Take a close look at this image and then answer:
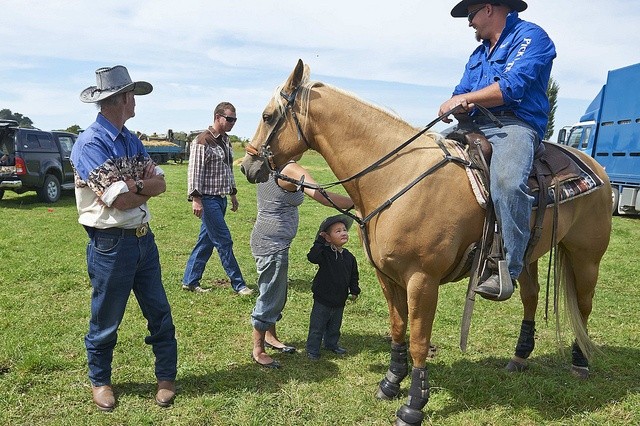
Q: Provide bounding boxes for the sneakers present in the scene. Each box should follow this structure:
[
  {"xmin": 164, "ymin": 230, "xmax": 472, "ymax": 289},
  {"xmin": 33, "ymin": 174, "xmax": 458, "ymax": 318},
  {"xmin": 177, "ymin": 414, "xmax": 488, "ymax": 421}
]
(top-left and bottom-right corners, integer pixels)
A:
[
  {"xmin": 183, "ymin": 285, "xmax": 207, "ymax": 294},
  {"xmin": 237, "ymin": 286, "xmax": 253, "ymax": 295}
]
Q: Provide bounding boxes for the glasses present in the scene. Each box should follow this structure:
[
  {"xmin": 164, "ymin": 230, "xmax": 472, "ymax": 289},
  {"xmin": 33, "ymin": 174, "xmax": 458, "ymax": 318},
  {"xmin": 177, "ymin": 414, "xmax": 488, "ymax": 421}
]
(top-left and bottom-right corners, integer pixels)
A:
[
  {"xmin": 467, "ymin": 4, "xmax": 499, "ymax": 23},
  {"xmin": 218, "ymin": 114, "xmax": 237, "ymax": 122}
]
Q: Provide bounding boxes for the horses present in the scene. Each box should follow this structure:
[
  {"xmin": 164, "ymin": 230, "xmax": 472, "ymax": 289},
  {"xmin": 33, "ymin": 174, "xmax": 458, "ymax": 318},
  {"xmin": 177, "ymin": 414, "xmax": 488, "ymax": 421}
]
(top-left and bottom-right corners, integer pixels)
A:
[{"xmin": 240, "ymin": 58, "xmax": 615, "ymax": 425}]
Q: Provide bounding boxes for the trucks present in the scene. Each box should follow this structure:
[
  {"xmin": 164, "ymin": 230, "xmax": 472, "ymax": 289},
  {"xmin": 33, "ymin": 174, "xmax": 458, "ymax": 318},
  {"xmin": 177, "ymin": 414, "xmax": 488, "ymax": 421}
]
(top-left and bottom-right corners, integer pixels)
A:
[
  {"xmin": 557, "ymin": 63, "xmax": 640, "ymax": 217},
  {"xmin": 144, "ymin": 146, "xmax": 183, "ymax": 165}
]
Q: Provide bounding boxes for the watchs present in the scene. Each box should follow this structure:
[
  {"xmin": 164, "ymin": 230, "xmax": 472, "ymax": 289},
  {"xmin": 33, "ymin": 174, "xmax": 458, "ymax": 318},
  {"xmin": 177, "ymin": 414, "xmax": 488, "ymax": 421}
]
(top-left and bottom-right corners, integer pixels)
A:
[{"xmin": 136, "ymin": 180, "xmax": 144, "ymax": 193}]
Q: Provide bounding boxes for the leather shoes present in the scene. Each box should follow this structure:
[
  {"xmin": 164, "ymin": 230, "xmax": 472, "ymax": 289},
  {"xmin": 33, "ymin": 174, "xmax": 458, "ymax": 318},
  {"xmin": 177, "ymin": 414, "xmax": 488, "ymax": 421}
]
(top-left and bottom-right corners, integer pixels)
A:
[
  {"xmin": 155, "ymin": 379, "xmax": 176, "ymax": 406},
  {"xmin": 474, "ymin": 273, "xmax": 515, "ymax": 297},
  {"xmin": 264, "ymin": 341, "xmax": 295, "ymax": 353},
  {"xmin": 331, "ymin": 346, "xmax": 346, "ymax": 354},
  {"xmin": 91, "ymin": 382, "xmax": 115, "ymax": 411},
  {"xmin": 307, "ymin": 352, "xmax": 321, "ymax": 360},
  {"xmin": 251, "ymin": 352, "xmax": 280, "ymax": 369}
]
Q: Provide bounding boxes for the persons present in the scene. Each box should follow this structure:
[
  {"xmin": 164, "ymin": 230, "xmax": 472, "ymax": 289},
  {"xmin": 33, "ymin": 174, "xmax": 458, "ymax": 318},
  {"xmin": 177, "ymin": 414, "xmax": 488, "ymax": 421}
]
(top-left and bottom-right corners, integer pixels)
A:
[
  {"xmin": 182, "ymin": 102, "xmax": 255, "ymax": 296},
  {"xmin": 250, "ymin": 151, "xmax": 354, "ymax": 368},
  {"xmin": 70, "ymin": 65, "xmax": 178, "ymax": 411},
  {"xmin": 438, "ymin": 0, "xmax": 557, "ymax": 297},
  {"xmin": 306, "ymin": 214, "xmax": 361, "ymax": 359}
]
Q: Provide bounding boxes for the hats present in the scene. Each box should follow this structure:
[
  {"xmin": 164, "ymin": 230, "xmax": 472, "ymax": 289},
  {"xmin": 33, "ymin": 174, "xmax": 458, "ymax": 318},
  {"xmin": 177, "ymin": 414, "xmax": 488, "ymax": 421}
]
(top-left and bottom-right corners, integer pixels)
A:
[
  {"xmin": 79, "ymin": 64, "xmax": 153, "ymax": 103},
  {"xmin": 315, "ymin": 214, "xmax": 353, "ymax": 247},
  {"xmin": 451, "ymin": 0, "xmax": 527, "ymax": 17}
]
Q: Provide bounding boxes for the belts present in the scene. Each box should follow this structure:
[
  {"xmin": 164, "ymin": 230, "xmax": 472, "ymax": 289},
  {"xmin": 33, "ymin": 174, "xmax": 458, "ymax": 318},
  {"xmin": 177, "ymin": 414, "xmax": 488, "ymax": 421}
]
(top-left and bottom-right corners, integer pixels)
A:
[
  {"xmin": 86, "ymin": 222, "xmax": 149, "ymax": 238},
  {"xmin": 202, "ymin": 194, "xmax": 226, "ymax": 198},
  {"xmin": 476, "ymin": 111, "xmax": 516, "ymax": 116}
]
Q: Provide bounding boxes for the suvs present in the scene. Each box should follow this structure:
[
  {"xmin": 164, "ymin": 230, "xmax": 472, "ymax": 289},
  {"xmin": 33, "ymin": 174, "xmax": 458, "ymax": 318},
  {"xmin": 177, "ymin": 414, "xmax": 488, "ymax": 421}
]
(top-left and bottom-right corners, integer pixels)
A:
[{"xmin": 1, "ymin": 119, "xmax": 78, "ymax": 202}]
